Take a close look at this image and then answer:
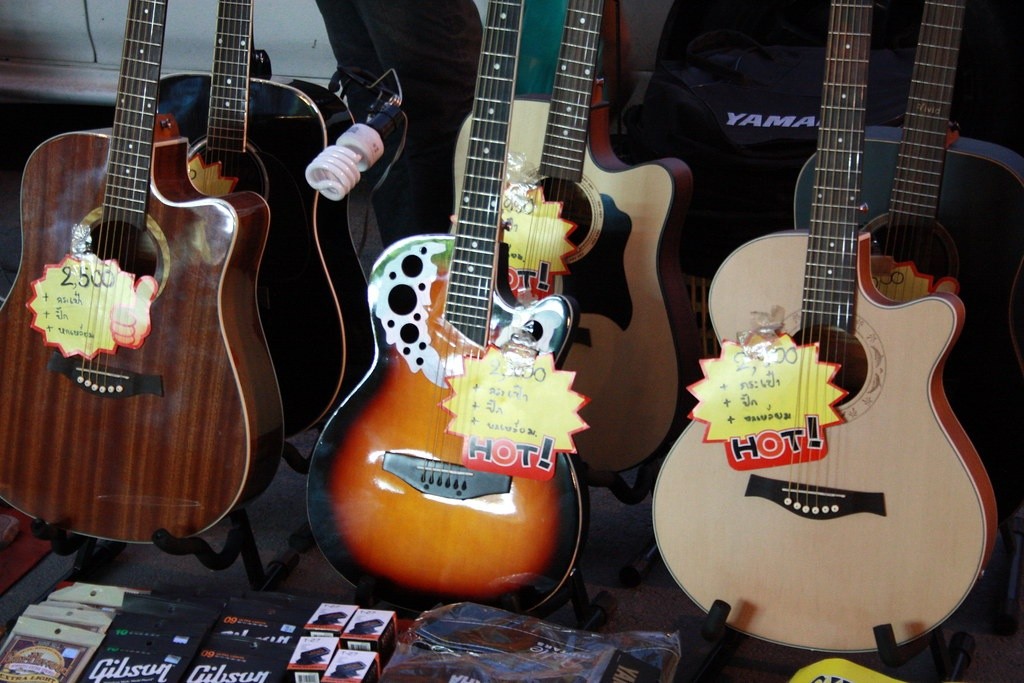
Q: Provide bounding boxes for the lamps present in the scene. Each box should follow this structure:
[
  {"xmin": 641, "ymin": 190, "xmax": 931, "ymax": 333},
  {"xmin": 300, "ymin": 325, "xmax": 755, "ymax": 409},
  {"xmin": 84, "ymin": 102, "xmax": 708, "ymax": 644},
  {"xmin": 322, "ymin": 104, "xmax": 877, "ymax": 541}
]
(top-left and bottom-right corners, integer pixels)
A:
[{"xmin": 305, "ymin": 73, "xmax": 400, "ymax": 201}]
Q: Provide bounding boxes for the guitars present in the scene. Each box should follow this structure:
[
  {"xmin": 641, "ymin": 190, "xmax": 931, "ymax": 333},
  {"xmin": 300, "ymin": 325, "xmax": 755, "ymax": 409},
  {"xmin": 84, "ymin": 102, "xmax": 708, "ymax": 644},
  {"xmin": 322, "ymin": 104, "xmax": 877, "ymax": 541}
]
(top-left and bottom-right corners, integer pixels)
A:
[
  {"xmin": 0, "ymin": 2, "xmax": 287, "ymax": 548},
  {"xmin": 789, "ymin": 1, "xmax": 1023, "ymax": 522},
  {"xmin": 303, "ymin": 0, "xmax": 590, "ymax": 617},
  {"xmin": 449, "ymin": 0, "xmax": 706, "ymax": 473},
  {"xmin": 649, "ymin": 0, "xmax": 1001, "ymax": 655},
  {"xmin": 158, "ymin": 1, "xmax": 376, "ymax": 443}
]
